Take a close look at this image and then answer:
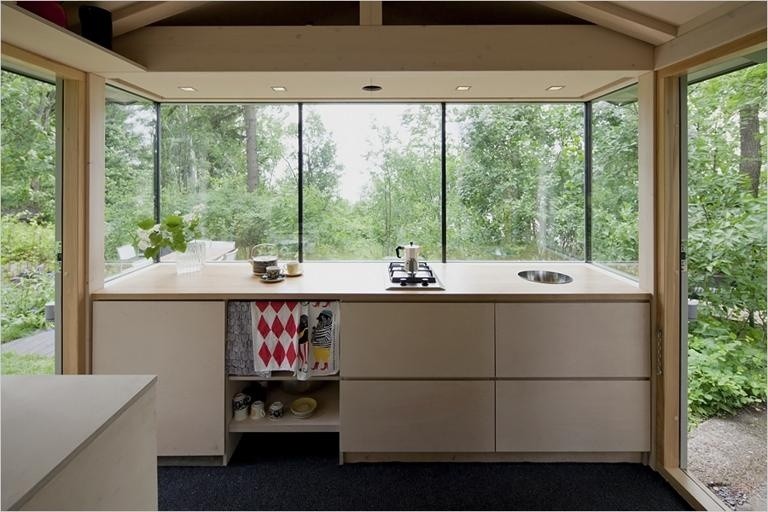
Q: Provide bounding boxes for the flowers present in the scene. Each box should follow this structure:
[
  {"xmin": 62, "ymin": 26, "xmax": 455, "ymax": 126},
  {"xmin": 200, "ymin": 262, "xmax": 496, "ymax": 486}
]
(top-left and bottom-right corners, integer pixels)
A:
[{"xmin": 135, "ymin": 204, "xmax": 209, "ymax": 264}]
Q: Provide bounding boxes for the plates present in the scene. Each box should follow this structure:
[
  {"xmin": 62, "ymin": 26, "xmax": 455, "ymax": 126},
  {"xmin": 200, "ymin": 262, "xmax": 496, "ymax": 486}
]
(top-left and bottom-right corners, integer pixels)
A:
[
  {"xmin": 279, "ymin": 268, "xmax": 304, "ymax": 276},
  {"xmin": 259, "ymin": 277, "xmax": 284, "ymax": 283},
  {"xmin": 288, "ymin": 410, "xmax": 316, "ymax": 419}
]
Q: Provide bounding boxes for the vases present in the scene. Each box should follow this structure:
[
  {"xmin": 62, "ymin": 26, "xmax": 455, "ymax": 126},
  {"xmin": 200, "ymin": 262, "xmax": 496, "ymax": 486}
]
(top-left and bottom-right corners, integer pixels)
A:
[{"xmin": 170, "ymin": 247, "xmax": 203, "ymax": 274}]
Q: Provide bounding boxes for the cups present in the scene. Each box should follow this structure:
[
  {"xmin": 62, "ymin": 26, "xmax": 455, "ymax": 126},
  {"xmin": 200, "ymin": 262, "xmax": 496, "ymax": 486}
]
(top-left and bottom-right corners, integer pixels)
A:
[
  {"xmin": 233, "ymin": 392, "xmax": 251, "ymax": 408},
  {"xmin": 267, "ymin": 402, "xmax": 286, "ymax": 422},
  {"xmin": 266, "ymin": 265, "xmax": 283, "ymax": 279},
  {"xmin": 233, "ymin": 405, "xmax": 249, "ymax": 422},
  {"xmin": 249, "ymin": 402, "xmax": 266, "ymax": 421},
  {"xmin": 283, "ymin": 261, "xmax": 300, "ymax": 274}
]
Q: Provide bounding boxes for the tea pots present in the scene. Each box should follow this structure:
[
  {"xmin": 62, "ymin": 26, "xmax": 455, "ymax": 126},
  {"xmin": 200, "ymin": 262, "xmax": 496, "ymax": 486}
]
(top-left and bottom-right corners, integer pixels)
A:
[{"xmin": 248, "ymin": 243, "xmax": 279, "ymax": 276}]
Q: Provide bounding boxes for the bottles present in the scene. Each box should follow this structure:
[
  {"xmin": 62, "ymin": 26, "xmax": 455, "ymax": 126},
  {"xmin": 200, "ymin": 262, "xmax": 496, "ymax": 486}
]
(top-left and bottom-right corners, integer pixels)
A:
[{"xmin": 395, "ymin": 242, "xmax": 423, "ymax": 274}]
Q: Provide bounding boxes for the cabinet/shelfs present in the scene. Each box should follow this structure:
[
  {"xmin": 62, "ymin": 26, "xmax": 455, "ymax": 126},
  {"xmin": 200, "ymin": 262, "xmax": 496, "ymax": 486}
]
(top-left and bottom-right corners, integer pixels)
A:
[
  {"xmin": 342, "ymin": 295, "xmax": 495, "ymax": 467},
  {"xmin": 225, "ymin": 294, "xmax": 341, "ymax": 434},
  {"xmin": 92, "ymin": 298, "xmax": 225, "ymax": 470},
  {"xmin": 496, "ymin": 297, "xmax": 653, "ymax": 466}
]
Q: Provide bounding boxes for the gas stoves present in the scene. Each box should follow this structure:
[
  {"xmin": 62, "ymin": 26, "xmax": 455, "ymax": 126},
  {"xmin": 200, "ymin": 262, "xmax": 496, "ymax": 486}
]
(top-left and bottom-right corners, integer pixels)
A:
[{"xmin": 384, "ymin": 258, "xmax": 446, "ymax": 292}]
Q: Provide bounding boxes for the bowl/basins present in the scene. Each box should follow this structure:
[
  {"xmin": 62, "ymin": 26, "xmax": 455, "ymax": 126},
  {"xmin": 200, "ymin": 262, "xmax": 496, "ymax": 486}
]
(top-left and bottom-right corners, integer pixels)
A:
[{"xmin": 290, "ymin": 397, "xmax": 318, "ymax": 419}]
